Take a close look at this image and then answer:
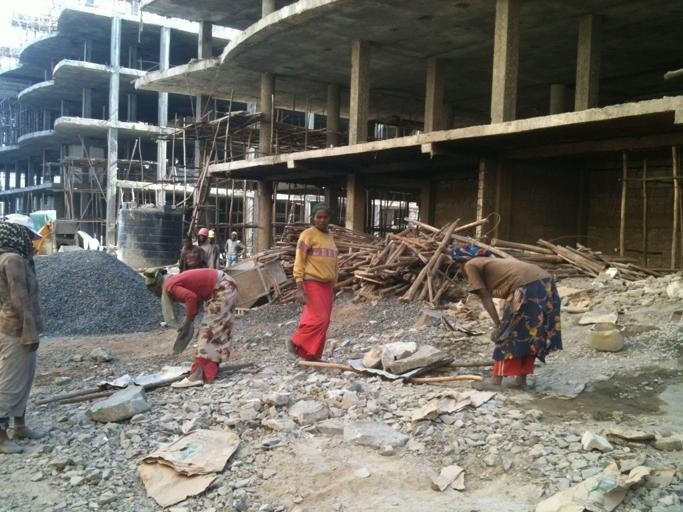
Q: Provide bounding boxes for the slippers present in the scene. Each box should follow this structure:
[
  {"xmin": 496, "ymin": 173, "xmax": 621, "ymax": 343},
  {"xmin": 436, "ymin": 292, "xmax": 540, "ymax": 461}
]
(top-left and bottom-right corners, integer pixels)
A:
[{"xmin": 170, "ymin": 377, "xmax": 203, "ymax": 388}]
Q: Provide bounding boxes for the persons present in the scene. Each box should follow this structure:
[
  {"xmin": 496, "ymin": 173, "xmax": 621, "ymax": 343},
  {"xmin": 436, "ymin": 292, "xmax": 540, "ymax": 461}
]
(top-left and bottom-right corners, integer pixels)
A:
[
  {"xmin": 144, "ymin": 267, "xmax": 238, "ymax": 388},
  {"xmin": 208, "ymin": 229, "xmax": 220, "ymax": 269},
  {"xmin": 284, "ymin": 202, "xmax": 339, "ymax": 363},
  {"xmin": 224, "ymin": 231, "xmax": 245, "ymax": 268},
  {"xmin": 0, "ymin": 213, "xmax": 46, "ymax": 454},
  {"xmin": 450, "ymin": 243, "xmax": 563, "ymax": 393},
  {"xmin": 178, "ymin": 235, "xmax": 208, "ymax": 273},
  {"xmin": 193, "ymin": 228, "xmax": 215, "ymax": 269}
]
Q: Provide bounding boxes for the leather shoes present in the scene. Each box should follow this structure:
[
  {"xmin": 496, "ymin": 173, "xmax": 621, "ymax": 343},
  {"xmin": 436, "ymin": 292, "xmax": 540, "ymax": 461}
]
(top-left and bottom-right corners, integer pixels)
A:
[
  {"xmin": 0, "ymin": 443, "xmax": 23, "ymax": 454},
  {"xmin": 14, "ymin": 429, "xmax": 44, "ymax": 439},
  {"xmin": 285, "ymin": 336, "xmax": 296, "ymax": 360}
]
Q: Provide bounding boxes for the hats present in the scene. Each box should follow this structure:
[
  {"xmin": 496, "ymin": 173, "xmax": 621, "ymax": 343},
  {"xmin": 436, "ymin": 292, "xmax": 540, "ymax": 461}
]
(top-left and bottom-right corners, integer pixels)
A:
[{"xmin": 4, "ymin": 213, "xmax": 42, "ymax": 240}]
